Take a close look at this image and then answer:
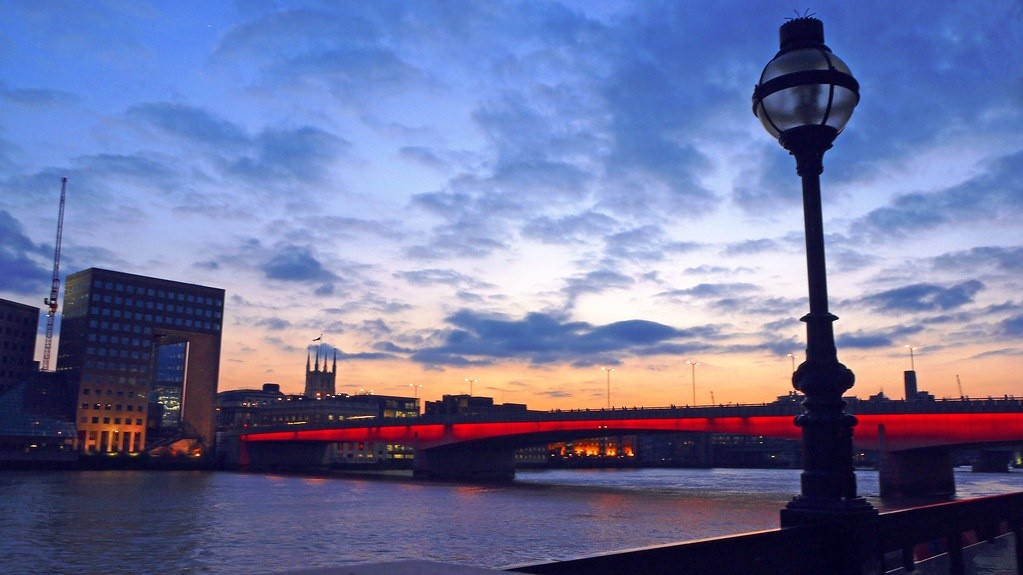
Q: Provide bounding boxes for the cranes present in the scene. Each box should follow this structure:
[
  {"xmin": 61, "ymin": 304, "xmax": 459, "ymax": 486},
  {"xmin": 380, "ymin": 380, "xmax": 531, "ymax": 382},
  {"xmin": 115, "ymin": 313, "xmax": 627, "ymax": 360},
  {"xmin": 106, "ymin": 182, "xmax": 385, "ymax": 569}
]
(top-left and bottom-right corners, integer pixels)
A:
[{"xmin": 41, "ymin": 176, "xmax": 68, "ymax": 370}]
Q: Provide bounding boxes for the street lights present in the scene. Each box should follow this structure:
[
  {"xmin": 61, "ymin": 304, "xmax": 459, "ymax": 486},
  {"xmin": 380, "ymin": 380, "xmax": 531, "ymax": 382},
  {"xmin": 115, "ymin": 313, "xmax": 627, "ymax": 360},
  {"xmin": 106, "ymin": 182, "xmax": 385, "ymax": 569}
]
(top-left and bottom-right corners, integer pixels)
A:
[
  {"xmin": 752, "ymin": 7, "xmax": 881, "ymax": 530},
  {"xmin": 787, "ymin": 353, "xmax": 798, "ymax": 373},
  {"xmin": 464, "ymin": 378, "xmax": 478, "ymax": 396},
  {"xmin": 602, "ymin": 367, "xmax": 615, "ymax": 411},
  {"xmin": 410, "ymin": 384, "xmax": 423, "ymax": 398},
  {"xmin": 686, "ymin": 360, "xmax": 701, "ymax": 408},
  {"xmin": 904, "ymin": 344, "xmax": 918, "ymax": 370}
]
